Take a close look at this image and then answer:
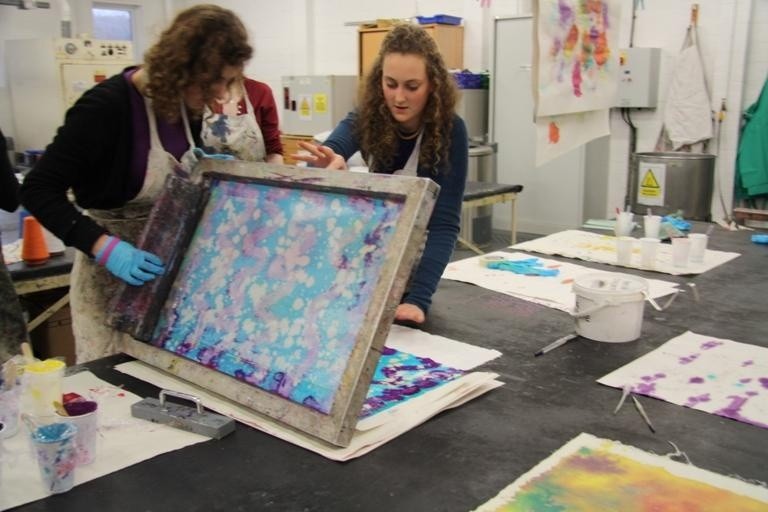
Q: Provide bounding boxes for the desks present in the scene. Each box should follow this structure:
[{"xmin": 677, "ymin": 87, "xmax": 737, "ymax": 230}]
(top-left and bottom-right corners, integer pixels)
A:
[{"xmin": 0, "ymin": 182, "xmax": 768, "ymax": 512}]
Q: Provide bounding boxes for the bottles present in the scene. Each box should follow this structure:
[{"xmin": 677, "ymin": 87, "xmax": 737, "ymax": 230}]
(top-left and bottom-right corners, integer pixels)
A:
[{"xmin": 15, "ymin": 208, "xmax": 65, "ymax": 266}]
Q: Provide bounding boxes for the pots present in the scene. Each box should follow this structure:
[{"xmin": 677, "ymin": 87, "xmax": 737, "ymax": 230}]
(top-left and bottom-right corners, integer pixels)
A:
[{"xmin": 626, "ymin": 150, "xmax": 716, "ymax": 221}]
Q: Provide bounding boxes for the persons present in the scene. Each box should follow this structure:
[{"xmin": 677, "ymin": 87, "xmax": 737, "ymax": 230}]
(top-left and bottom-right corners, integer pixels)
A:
[
  {"xmin": 0, "ymin": 131, "xmax": 22, "ymax": 213},
  {"xmin": 288, "ymin": 23, "xmax": 469, "ymax": 325},
  {"xmin": 198, "ymin": 74, "xmax": 284, "ymax": 166},
  {"xmin": 21, "ymin": 3, "xmax": 255, "ymax": 367}
]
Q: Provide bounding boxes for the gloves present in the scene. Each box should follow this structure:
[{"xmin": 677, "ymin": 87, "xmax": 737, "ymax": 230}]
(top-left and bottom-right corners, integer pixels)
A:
[
  {"xmin": 489, "ymin": 257, "xmax": 559, "ymax": 277},
  {"xmin": 95, "ymin": 238, "xmax": 165, "ymax": 286}
]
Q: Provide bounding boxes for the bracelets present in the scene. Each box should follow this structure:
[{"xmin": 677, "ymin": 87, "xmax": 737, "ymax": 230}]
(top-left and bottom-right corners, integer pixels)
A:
[{"xmin": 97, "ymin": 235, "xmax": 121, "ymax": 266}]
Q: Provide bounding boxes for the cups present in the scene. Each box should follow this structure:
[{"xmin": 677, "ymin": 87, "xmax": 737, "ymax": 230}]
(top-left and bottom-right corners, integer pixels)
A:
[
  {"xmin": 56, "ymin": 399, "xmax": 99, "ymax": 465},
  {"xmin": 642, "ymin": 214, "xmax": 661, "ymax": 239},
  {"xmin": 0, "ymin": 373, "xmax": 24, "ymax": 437},
  {"xmin": 31, "ymin": 421, "xmax": 78, "ymax": 496},
  {"xmin": 669, "ymin": 237, "xmax": 693, "ymax": 269},
  {"xmin": 686, "ymin": 233, "xmax": 709, "ymax": 263},
  {"xmin": 614, "ymin": 235, "xmax": 636, "ymax": 265},
  {"xmin": 23, "ymin": 360, "xmax": 69, "ymax": 417},
  {"xmin": 614, "ymin": 212, "xmax": 634, "ymax": 236},
  {"xmin": 637, "ymin": 237, "xmax": 662, "ymax": 269}
]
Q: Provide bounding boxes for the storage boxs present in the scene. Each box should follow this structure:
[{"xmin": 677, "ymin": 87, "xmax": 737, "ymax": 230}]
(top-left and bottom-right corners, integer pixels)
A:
[{"xmin": 356, "ymin": 25, "xmax": 463, "ymax": 79}]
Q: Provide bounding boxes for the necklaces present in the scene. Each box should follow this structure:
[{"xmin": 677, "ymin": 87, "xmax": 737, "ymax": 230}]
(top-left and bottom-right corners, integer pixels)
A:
[{"xmin": 393, "ymin": 124, "xmax": 422, "ymax": 140}]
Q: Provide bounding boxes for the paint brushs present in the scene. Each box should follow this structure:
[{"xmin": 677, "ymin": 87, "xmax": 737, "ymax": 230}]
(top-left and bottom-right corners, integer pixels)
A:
[
  {"xmin": 631, "ymin": 395, "xmax": 656, "ymax": 434},
  {"xmin": 613, "ymin": 384, "xmax": 634, "ymax": 416},
  {"xmin": 534, "ymin": 333, "xmax": 580, "ymax": 357}
]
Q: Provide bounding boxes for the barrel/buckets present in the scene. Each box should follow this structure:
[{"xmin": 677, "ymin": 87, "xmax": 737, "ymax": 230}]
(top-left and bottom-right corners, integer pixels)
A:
[
  {"xmin": 626, "ymin": 150, "xmax": 719, "ymax": 221},
  {"xmin": 566, "ymin": 275, "xmax": 648, "ymax": 343}
]
